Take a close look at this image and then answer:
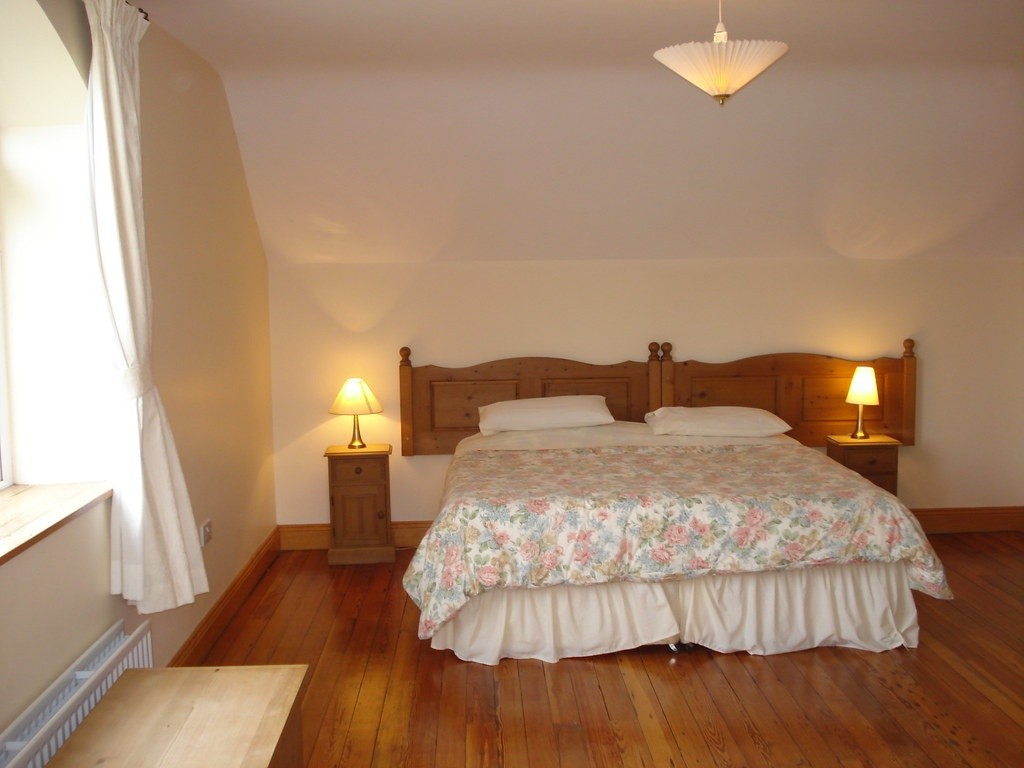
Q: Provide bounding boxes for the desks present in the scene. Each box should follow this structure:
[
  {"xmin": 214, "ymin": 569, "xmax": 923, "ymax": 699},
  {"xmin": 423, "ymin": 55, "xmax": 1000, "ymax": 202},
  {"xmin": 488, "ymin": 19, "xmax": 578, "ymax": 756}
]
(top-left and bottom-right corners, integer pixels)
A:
[{"xmin": 43, "ymin": 662, "xmax": 314, "ymax": 768}]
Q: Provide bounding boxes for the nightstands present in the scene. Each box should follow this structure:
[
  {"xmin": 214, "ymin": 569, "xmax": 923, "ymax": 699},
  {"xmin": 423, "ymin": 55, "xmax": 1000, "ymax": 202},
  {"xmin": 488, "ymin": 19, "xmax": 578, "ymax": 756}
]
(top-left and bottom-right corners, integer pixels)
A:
[
  {"xmin": 824, "ymin": 435, "xmax": 903, "ymax": 496},
  {"xmin": 322, "ymin": 443, "xmax": 395, "ymax": 565}
]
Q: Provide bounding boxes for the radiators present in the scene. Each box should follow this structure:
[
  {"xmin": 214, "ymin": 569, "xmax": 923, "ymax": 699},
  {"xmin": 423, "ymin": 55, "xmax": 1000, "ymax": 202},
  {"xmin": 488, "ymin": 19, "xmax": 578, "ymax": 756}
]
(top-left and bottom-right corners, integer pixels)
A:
[{"xmin": 0, "ymin": 619, "xmax": 154, "ymax": 767}]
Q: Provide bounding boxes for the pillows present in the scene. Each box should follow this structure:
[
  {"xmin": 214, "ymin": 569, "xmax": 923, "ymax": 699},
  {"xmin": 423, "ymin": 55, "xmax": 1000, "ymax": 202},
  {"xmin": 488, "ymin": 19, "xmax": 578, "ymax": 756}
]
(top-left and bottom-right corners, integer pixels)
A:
[
  {"xmin": 644, "ymin": 405, "xmax": 794, "ymax": 436},
  {"xmin": 478, "ymin": 394, "xmax": 615, "ymax": 437}
]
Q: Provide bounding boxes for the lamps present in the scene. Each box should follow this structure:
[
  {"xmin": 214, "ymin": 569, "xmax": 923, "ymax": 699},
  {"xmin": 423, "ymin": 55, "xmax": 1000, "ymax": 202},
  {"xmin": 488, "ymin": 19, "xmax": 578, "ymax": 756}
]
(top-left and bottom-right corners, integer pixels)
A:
[
  {"xmin": 329, "ymin": 377, "xmax": 383, "ymax": 449},
  {"xmin": 843, "ymin": 366, "xmax": 881, "ymax": 439},
  {"xmin": 653, "ymin": 1, "xmax": 791, "ymax": 107}
]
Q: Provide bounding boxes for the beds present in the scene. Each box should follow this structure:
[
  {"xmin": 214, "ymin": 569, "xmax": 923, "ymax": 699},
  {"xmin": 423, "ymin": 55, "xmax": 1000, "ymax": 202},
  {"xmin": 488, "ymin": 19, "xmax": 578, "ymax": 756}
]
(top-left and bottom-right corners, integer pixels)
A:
[{"xmin": 398, "ymin": 338, "xmax": 953, "ymax": 666}]
